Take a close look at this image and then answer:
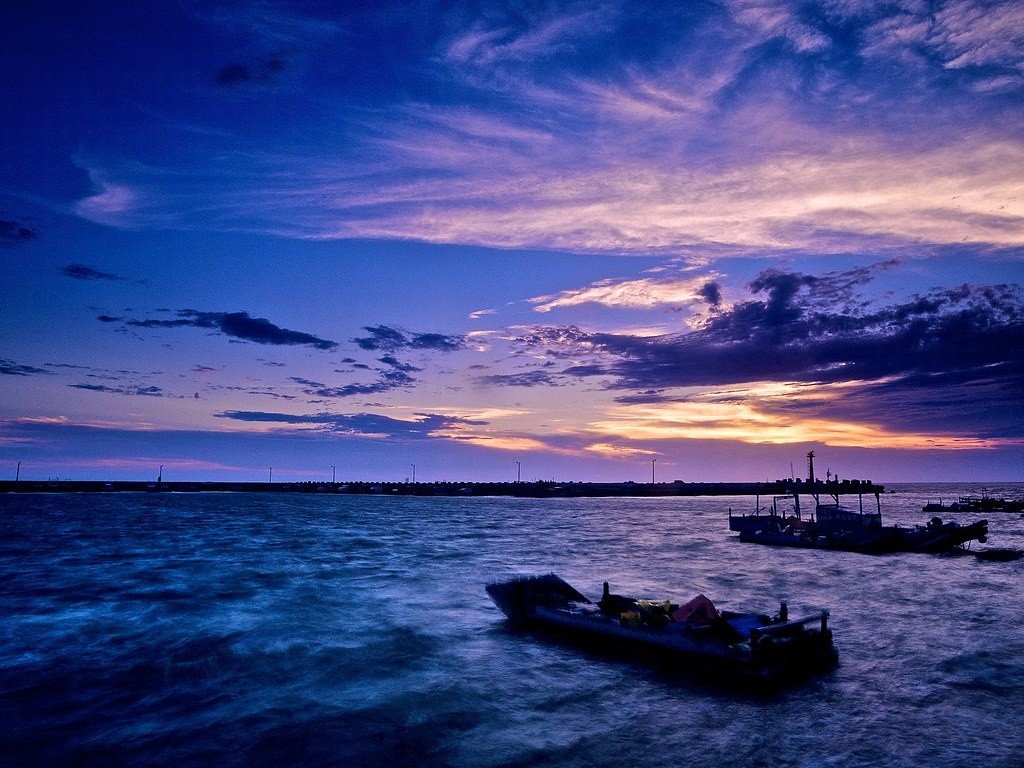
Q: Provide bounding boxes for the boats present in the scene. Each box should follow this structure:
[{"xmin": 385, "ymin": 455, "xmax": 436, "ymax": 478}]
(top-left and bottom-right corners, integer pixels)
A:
[
  {"xmin": 922, "ymin": 492, "xmax": 1024, "ymax": 513},
  {"xmin": 484, "ymin": 571, "xmax": 841, "ymax": 691},
  {"xmin": 728, "ymin": 484, "xmax": 988, "ymax": 556}
]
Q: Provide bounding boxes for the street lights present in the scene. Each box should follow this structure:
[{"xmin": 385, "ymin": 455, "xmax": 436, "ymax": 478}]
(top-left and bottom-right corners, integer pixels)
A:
[
  {"xmin": 159, "ymin": 464, "xmax": 164, "ymax": 482},
  {"xmin": 269, "ymin": 466, "xmax": 272, "ymax": 483},
  {"xmin": 331, "ymin": 465, "xmax": 336, "ymax": 482},
  {"xmin": 652, "ymin": 459, "xmax": 656, "ymax": 483},
  {"xmin": 515, "ymin": 461, "xmax": 521, "ymax": 483},
  {"xmin": 16, "ymin": 462, "xmax": 22, "ymax": 480},
  {"xmin": 411, "ymin": 463, "xmax": 415, "ymax": 483}
]
